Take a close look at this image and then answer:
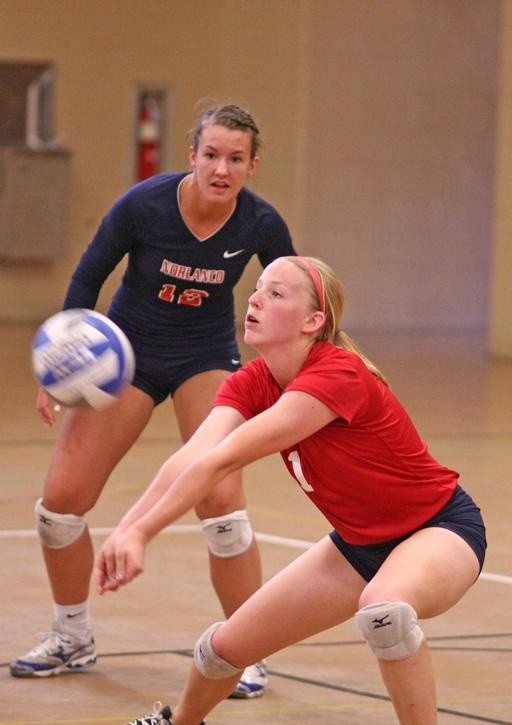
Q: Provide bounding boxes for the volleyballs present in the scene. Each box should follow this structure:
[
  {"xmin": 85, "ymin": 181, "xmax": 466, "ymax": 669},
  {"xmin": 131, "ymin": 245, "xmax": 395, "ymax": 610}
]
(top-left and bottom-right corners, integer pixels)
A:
[{"xmin": 31, "ymin": 308, "xmax": 135, "ymax": 410}]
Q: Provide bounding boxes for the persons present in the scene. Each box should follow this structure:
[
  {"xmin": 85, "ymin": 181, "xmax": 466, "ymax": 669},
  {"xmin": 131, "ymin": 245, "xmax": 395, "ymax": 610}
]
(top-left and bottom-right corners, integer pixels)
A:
[
  {"xmin": 9, "ymin": 105, "xmax": 299, "ymax": 697},
  {"xmin": 94, "ymin": 253, "xmax": 488, "ymax": 725}
]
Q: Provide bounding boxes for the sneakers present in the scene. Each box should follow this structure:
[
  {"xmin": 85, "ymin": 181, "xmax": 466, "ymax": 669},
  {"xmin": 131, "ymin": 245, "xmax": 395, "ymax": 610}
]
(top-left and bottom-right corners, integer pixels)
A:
[
  {"xmin": 9, "ymin": 631, "xmax": 96, "ymax": 678},
  {"xmin": 125, "ymin": 700, "xmax": 206, "ymax": 723},
  {"xmin": 227, "ymin": 658, "xmax": 267, "ymax": 697}
]
